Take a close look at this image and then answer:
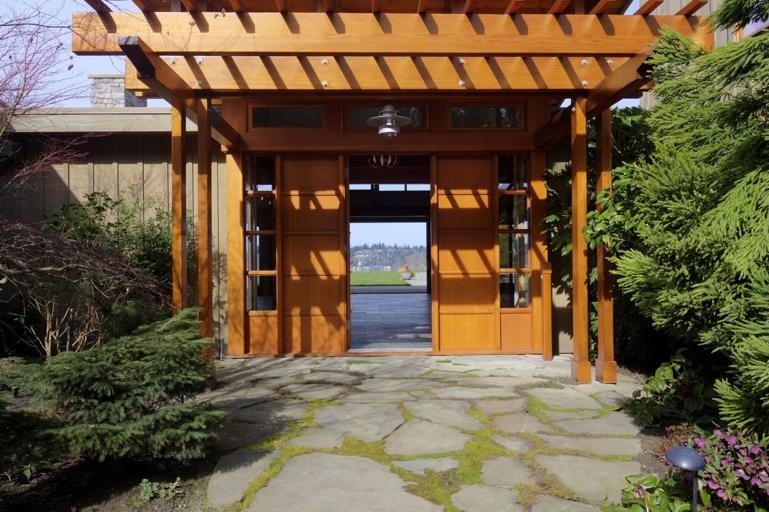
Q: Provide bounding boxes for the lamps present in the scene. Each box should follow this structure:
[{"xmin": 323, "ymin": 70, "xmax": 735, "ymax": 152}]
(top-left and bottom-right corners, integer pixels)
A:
[{"xmin": 365, "ymin": 104, "xmax": 412, "ymax": 138}]
